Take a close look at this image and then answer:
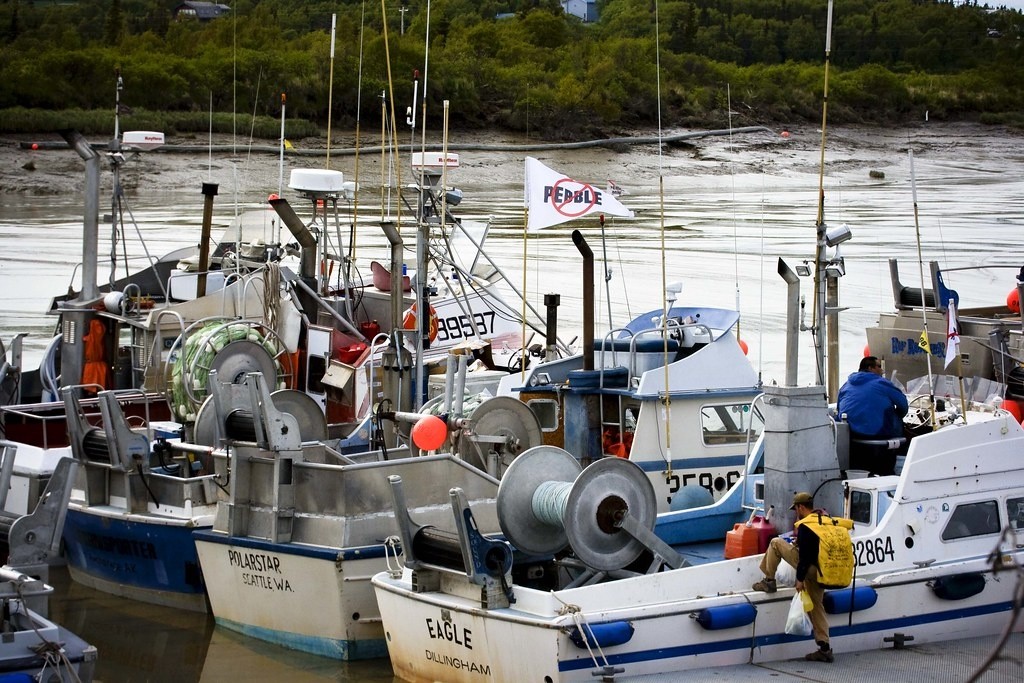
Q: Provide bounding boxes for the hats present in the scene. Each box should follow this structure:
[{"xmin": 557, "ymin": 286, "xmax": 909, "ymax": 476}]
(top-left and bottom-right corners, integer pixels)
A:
[{"xmin": 789, "ymin": 493, "xmax": 813, "ymax": 510}]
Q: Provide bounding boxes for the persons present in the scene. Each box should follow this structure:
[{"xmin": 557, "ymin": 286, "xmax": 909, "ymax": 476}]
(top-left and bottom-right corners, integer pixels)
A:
[
  {"xmin": 838, "ymin": 355, "xmax": 909, "ymax": 477},
  {"xmin": 753, "ymin": 492, "xmax": 835, "ymax": 663}
]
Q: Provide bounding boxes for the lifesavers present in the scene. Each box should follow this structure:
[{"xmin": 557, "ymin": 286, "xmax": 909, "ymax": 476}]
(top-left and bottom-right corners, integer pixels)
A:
[{"xmin": 402, "ymin": 297, "xmax": 440, "ymax": 348}]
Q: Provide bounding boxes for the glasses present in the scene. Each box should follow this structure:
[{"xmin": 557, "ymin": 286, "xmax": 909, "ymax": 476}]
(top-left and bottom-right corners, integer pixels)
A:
[{"xmin": 876, "ymin": 364, "xmax": 881, "ymax": 368}]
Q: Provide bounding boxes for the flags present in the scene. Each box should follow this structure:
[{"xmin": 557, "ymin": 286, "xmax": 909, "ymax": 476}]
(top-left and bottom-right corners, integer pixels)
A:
[
  {"xmin": 606, "ymin": 178, "xmax": 629, "ymax": 197},
  {"xmin": 945, "ymin": 298, "xmax": 960, "ymax": 369},
  {"xmin": 524, "ymin": 159, "xmax": 631, "ymax": 231}
]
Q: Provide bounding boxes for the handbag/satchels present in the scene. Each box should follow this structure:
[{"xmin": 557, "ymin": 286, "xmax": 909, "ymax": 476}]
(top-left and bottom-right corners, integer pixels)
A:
[{"xmin": 784, "ymin": 589, "xmax": 814, "ymax": 636}]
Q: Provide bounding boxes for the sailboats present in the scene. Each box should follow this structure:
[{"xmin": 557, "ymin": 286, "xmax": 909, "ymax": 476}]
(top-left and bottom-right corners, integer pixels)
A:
[{"xmin": 0, "ymin": 1, "xmax": 1024, "ymax": 683}]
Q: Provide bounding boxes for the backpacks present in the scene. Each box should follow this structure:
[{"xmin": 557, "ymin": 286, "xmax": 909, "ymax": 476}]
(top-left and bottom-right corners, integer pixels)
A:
[{"xmin": 794, "ymin": 513, "xmax": 855, "ymax": 589}]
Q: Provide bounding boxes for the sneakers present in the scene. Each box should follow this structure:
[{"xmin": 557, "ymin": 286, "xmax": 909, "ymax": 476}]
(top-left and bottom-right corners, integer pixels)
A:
[
  {"xmin": 752, "ymin": 579, "xmax": 776, "ymax": 593},
  {"xmin": 805, "ymin": 648, "xmax": 834, "ymax": 663}
]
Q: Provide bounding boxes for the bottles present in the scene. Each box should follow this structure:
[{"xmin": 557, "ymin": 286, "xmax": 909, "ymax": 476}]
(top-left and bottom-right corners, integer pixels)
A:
[
  {"xmin": 753, "ymin": 516, "xmax": 777, "ymax": 553},
  {"xmin": 403, "ymin": 264, "xmax": 407, "ymax": 275},
  {"xmin": 725, "ymin": 524, "xmax": 759, "ymax": 559}
]
